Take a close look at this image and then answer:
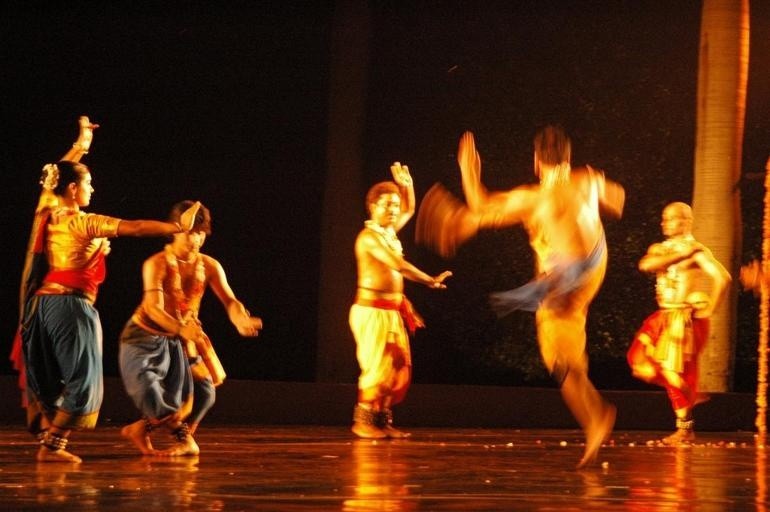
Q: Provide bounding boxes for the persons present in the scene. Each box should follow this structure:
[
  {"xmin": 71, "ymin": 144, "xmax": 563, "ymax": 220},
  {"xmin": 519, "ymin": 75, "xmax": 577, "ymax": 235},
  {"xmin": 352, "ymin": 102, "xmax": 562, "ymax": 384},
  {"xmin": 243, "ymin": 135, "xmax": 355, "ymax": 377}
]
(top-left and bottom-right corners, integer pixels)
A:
[
  {"xmin": 11, "ymin": 116, "xmax": 201, "ymax": 463},
  {"xmin": 403, "ymin": 122, "xmax": 630, "ymax": 471},
  {"xmin": 119, "ymin": 198, "xmax": 264, "ymax": 456},
  {"xmin": 627, "ymin": 200, "xmax": 734, "ymax": 444},
  {"xmin": 348, "ymin": 160, "xmax": 454, "ymax": 440}
]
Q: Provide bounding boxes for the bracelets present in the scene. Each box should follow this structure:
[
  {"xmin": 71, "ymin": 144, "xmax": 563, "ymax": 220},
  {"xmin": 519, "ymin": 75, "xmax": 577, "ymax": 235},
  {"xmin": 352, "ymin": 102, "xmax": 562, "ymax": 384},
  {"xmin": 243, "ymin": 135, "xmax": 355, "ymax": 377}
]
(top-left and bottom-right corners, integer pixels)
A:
[
  {"xmin": 175, "ymin": 221, "xmax": 187, "ymax": 234},
  {"xmin": 72, "ymin": 143, "xmax": 89, "ymax": 155}
]
